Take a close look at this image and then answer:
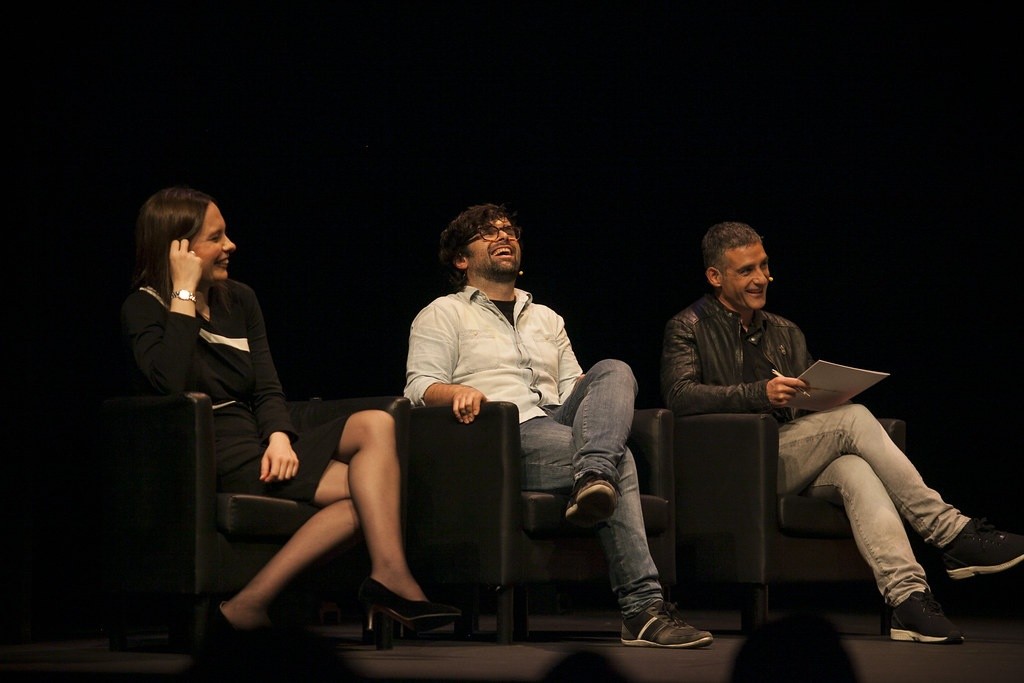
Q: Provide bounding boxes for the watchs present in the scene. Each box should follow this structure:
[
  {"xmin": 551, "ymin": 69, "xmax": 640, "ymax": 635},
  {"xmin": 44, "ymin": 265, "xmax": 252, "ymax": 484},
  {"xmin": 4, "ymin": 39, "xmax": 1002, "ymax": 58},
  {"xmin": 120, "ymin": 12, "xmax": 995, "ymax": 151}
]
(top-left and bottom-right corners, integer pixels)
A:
[{"xmin": 169, "ymin": 288, "xmax": 198, "ymax": 303}]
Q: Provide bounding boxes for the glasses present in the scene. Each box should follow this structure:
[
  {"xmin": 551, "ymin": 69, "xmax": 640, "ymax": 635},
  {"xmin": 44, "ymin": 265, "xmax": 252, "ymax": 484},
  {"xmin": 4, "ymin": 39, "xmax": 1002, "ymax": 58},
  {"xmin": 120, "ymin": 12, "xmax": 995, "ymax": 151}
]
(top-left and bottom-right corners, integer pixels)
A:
[{"xmin": 459, "ymin": 224, "xmax": 522, "ymax": 241}]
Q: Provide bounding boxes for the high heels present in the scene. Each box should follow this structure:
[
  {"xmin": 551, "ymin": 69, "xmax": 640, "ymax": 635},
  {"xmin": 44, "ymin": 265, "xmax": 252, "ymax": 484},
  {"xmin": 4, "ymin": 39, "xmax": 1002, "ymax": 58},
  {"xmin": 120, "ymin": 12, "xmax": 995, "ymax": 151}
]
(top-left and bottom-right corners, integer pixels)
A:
[
  {"xmin": 357, "ymin": 577, "xmax": 464, "ymax": 635},
  {"xmin": 212, "ymin": 602, "xmax": 239, "ymax": 637}
]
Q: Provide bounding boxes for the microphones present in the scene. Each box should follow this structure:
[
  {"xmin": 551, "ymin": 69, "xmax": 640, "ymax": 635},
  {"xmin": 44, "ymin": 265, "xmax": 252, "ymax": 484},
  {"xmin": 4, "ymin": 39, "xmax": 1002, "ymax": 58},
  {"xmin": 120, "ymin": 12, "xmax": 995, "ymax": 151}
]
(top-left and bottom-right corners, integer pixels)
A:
[
  {"xmin": 519, "ymin": 270, "xmax": 523, "ymax": 275},
  {"xmin": 769, "ymin": 277, "xmax": 773, "ymax": 282}
]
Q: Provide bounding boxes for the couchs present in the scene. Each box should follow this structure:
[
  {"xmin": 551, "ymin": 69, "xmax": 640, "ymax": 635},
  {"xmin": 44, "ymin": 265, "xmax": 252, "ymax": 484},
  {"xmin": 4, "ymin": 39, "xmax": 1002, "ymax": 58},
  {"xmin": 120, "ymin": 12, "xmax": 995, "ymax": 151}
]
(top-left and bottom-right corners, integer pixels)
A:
[
  {"xmin": 360, "ymin": 408, "xmax": 680, "ymax": 647},
  {"xmin": 676, "ymin": 413, "xmax": 910, "ymax": 637},
  {"xmin": 106, "ymin": 394, "xmax": 411, "ymax": 652}
]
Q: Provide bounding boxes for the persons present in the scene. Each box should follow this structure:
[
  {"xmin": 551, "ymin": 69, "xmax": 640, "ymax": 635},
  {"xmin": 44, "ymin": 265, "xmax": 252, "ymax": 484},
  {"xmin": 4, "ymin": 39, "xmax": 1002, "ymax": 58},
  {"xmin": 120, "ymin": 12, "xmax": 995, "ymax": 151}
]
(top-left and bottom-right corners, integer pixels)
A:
[
  {"xmin": 526, "ymin": 636, "xmax": 642, "ymax": 683},
  {"xmin": 166, "ymin": 620, "xmax": 395, "ymax": 683},
  {"xmin": 662, "ymin": 221, "xmax": 1024, "ymax": 647},
  {"xmin": 401, "ymin": 201, "xmax": 714, "ymax": 649},
  {"xmin": 108, "ymin": 183, "xmax": 463, "ymax": 662},
  {"xmin": 721, "ymin": 605, "xmax": 868, "ymax": 683}
]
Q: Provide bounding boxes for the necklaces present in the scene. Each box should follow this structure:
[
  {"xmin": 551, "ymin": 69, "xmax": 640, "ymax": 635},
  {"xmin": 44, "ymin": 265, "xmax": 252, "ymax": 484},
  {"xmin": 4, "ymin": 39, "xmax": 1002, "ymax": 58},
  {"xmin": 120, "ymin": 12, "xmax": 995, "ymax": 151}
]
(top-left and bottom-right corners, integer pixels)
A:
[{"xmin": 188, "ymin": 296, "xmax": 213, "ymax": 321}]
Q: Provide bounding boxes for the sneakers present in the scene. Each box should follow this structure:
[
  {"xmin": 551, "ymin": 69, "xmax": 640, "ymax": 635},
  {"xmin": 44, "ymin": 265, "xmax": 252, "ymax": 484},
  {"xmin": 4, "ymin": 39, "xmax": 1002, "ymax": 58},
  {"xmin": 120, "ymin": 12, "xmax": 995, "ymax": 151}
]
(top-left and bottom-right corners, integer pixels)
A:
[
  {"xmin": 888, "ymin": 592, "xmax": 966, "ymax": 644},
  {"xmin": 564, "ymin": 473, "xmax": 617, "ymax": 529},
  {"xmin": 945, "ymin": 518, "xmax": 1024, "ymax": 580},
  {"xmin": 622, "ymin": 601, "xmax": 714, "ymax": 650}
]
